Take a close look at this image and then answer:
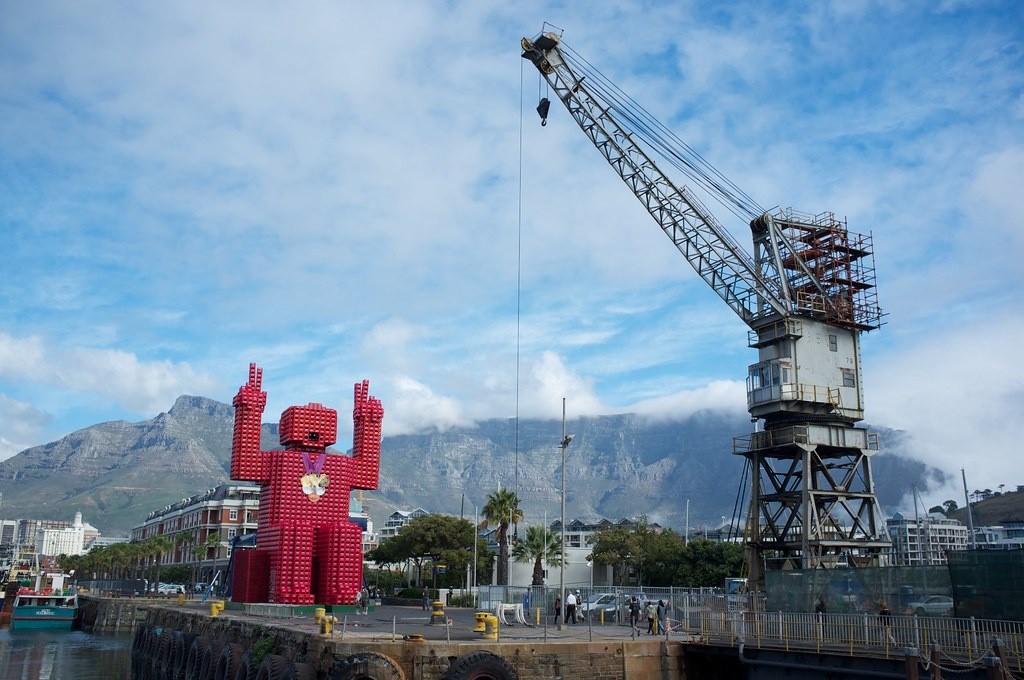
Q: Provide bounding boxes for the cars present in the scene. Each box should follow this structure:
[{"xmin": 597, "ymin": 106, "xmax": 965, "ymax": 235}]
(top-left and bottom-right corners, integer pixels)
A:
[
  {"xmin": 906, "ymin": 594, "xmax": 954, "ymax": 617},
  {"xmin": 579, "ymin": 592, "xmax": 670, "ymax": 623},
  {"xmin": 134, "ymin": 579, "xmax": 210, "ymax": 595}
]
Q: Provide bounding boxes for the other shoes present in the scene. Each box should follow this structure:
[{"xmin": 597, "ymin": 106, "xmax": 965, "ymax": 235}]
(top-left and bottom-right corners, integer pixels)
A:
[
  {"xmin": 663, "ymin": 630, "xmax": 665, "ymax": 635},
  {"xmin": 637, "ymin": 630, "xmax": 640, "ymax": 636},
  {"xmin": 631, "ymin": 634, "xmax": 633, "ymax": 637}
]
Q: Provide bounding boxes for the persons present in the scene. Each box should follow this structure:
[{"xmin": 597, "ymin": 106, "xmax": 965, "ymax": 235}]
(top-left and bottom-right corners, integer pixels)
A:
[
  {"xmin": 354, "ymin": 589, "xmax": 363, "ymax": 616},
  {"xmin": 627, "ymin": 596, "xmax": 640, "ymax": 638},
  {"xmin": 645, "ymin": 601, "xmax": 655, "ymax": 635},
  {"xmin": 657, "ymin": 600, "xmax": 666, "ymax": 635},
  {"xmin": 814, "ymin": 596, "xmax": 826, "ymax": 643},
  {"xmin": 360, "ymin": 587, "xmax": 370, "ymax": 615},
  {"xmin": 877, "ymin": 602, "xmax": 897, "ymax": 646},
  {"xmin": 575, "ymin": 589, "xmax": 584, "ymax": 624},
  {"xmin": 422, "ymin": 586, "xmax": 430, "ymax": 611},
  {"xmin": 184, "ymin": 581, "xmax": 227, "ymax": 600},
  {"xmin": 554, "ymin": 594, "xmax": 560, "ymax": 624},
  {"xmin": 564, "ymin": 590, "xmax": 576, "ymax": 625}
]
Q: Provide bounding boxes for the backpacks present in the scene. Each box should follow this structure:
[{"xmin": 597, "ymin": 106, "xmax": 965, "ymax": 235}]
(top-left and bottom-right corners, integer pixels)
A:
[{"xmin": 576, "ymin": 594, "xmax": 581, "ymax": 605}]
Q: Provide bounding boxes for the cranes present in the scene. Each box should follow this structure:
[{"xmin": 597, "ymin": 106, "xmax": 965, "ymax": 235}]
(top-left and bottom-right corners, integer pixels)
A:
[{"xmin": 517, "ymin": 19, "xmax": 899, "ymax": 594}]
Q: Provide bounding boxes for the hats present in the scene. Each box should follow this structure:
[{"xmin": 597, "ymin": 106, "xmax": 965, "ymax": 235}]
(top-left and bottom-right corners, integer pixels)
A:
[{"xmin": 576, "ymin": 590, "xmax": 580, "ymax": 594}]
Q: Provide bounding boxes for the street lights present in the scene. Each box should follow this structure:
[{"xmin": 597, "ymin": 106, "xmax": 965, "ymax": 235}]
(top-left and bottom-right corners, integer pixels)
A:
[{"xmin": 558, "ymin": 397, "xmax": 578, "ymax": 633}]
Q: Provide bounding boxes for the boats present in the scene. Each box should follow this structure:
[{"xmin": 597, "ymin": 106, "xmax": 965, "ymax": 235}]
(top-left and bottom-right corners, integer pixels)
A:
[{"xmin": 10, "ymin": 591, "xmax": 80, "ymax": 630}]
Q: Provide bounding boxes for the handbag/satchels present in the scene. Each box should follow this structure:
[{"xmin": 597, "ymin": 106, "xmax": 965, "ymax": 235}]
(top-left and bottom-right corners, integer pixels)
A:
[{"xmin": 555, "ymin": 600, "xmax": 560, "ymax": 609}]
